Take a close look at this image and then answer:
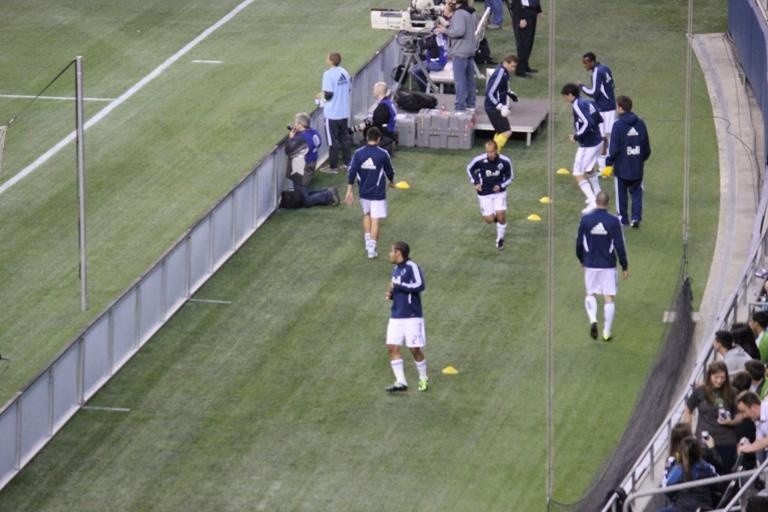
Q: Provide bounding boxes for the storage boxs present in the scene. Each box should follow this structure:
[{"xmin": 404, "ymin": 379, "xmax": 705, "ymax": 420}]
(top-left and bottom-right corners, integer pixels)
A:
[
  {"xmin": 416, "ymin": 108, "xmax": 475, "ymax": 150},
  {"xmin": 367, "ymin": 113, "xmax": 415, "ymax": 147}
]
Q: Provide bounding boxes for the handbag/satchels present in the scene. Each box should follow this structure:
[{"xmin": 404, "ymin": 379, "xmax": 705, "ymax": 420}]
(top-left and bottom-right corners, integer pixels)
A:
[{"xmin": 280, "ymin": 190, "xmax": 303, "ymax": 208}]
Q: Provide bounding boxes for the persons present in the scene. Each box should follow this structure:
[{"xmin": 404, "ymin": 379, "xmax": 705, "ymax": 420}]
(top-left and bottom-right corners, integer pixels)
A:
[
  {"xmin": 561, "ymin": 51, "xmax": 650, "ymax": 229},
  {"xmin": 349, "ymin": 81, "xmax": 397, "ymax": 151},
  {"xmin": 315, "ymin": 53, "xmax": 352, "ymax": 174},
  {"xmin": 410, "ymin": 1, "xmax": 543, "ymax": 113},
  {"xmin": 344, "ymin": 126, "xmax": 395, "ymax": 258},
  {"xmin": 484, "ymin": 55, "xmax": 520, "ymax": 153},
  {"xmin": 385, "ymin": 242, "xmax": 429, "ymax": 391},
  {"xmin": 285, "ymin": 112, "xmax": 340, "ymax": 207},
  {"xmin": 576, "ymin": 191, "xmax": 629, "ymax": 342},
  {"xmin": 466, "ymin": 140, "xmax": 514, "ymax": 250},
  {"xmin": 662, "ymin": 279, "xmax": 768, "ymax": 512}
]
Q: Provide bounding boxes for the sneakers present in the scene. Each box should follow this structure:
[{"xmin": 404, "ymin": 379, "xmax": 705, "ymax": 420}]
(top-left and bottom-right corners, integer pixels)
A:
[
  {"xmin": 590, "ymin": 321, "xmax": 598, "ymax": 340},
  {"xmin": 630, "ymin": 220, "xmax": 639, "ymax": 227},
  {"xmin": 324, "ymin": 186, "xmax": 340, "ymax": 207},
  {"xmin": 496, "ymin": 238, "xmax": 505, "ymax": 250},
  {"xmin": 319, "ymin": 165, "xmax": 349, "ymax": 174},
  {"xmin": 417, "ymin": 377, "xmax": 429, "ymax": 391},
  {"xmin": 385, "ymin": 381, "xmax": 408, "ymax": 392}
]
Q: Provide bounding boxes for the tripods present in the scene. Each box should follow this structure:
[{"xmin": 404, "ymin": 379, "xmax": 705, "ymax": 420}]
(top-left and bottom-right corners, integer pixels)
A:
[{"xmin": 386, "ymin": 51, "xmax": 440, "ymax": 94}]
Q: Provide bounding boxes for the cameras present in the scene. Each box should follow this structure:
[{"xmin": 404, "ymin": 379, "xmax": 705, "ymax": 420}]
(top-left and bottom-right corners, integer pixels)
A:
[{"xmin": 286, "ymin": 123, "xmax": 297, "ymax": 133}]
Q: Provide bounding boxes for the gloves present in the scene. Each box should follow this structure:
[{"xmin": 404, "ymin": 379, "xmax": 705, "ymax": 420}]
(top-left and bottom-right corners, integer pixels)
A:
[
  {"xmin": 509, "ymin": 90, "xmax": 519, "ymax": 102},
  {"xmin": 500, "ymin": 105, "xmax": 511, "ymax": 117}
]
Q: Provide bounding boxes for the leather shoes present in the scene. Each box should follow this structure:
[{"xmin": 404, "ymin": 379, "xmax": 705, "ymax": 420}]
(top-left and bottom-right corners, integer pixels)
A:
[{"xmin": 516, "ymin": 69, "xmax": 538, "ymax": 78}]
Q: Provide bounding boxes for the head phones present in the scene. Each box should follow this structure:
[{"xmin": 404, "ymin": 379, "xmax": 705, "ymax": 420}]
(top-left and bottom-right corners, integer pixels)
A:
[{"xmin": 448, "ymin": 0, "xmax": 461, "ymax": 9}]
